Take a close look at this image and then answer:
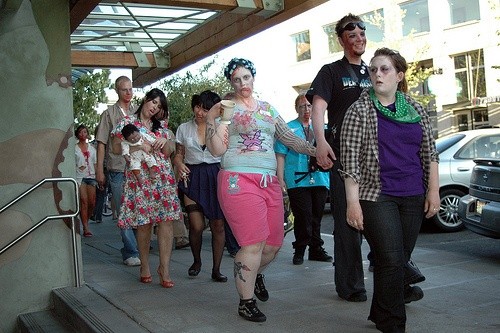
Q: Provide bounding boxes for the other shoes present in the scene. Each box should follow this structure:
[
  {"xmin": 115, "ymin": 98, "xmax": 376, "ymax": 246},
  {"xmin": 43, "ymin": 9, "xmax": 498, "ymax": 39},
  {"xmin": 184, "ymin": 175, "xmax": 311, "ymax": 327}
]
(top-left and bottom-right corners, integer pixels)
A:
[
  {"xmin": 407, "ymin": 260, "xmax": 425, "ymax": 284},
  {"xmin": 123, "ymin": 256, "xmax": 141, "ymax": 266},
  {"xmin": 188, "ymin": 263, "xmax": 201, "ymax": 276},
  {"xmin": 404, "ymin": 281, "xmax": 423, "ymax": 303},
  {"xmin": 83, "ymin": 232, "xmax": 92, "ymax": 237},
  {"xmin": 212, "ymin": 273, "xmax": 227, "ymax": 282},
  {"xmin": 338, "ymin": 290, "xmax": 366, "ymax": 302},
  {"xmin": 308, "ymin": 248, "xmax": 332, "ymax": 262},
  {"xmin": 293, "ymin": 249, "xmax": 304, "ymax": 264}
]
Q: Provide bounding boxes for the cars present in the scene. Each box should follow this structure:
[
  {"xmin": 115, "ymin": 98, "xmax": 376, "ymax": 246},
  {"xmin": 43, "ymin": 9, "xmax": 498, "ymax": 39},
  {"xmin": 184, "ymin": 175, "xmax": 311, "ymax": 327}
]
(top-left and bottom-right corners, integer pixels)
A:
[
  {"xmin": 435, "ymin": 128, "xmax": 498, "ymax": 233},
  {"xmin": 457, "ymin": 156, "xmax": 498, "ymax": 240}
]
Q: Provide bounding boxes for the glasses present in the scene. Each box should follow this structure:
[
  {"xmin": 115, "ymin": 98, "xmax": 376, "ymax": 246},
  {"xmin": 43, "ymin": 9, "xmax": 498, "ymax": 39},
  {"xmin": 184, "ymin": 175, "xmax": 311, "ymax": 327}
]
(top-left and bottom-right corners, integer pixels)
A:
[{"xmin": 341, "ymin": 22, "xmax": 365, "ymax": 36}]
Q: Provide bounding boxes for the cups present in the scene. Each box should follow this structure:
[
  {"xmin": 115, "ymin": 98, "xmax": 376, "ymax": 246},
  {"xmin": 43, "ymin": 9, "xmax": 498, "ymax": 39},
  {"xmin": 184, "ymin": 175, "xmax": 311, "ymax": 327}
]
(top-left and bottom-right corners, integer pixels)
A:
[{"xmin": 218, "ymin": 99, "xmax": 236, "ymax": 125}]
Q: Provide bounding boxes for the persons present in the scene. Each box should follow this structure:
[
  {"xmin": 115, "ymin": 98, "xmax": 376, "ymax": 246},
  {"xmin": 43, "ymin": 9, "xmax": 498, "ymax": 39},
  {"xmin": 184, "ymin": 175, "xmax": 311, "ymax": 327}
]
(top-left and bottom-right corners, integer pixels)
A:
[
  {"xmin": 337, "ymin": 49, "xmax": 441, "ymax": 333},
  {"xmin": 73, "ymin": 125, "xmax": 98, "ymax": 238},
  {"xmin": 120, "ymin": 123, "xmax": 157, "ymax": 180},
  {"xmin": 306, "ymin": 14, "xmax": 424, "ymax": 305},
  {"xmin": 91, "ymin": 76, "xmax": 240, "ymax": 287},
  {"xmin": 205, "ymin": 57, "xmax": 333, "ymax": 323},
  {"xmin": 274, "ymin": 93, "xmax": 333, "ymax": 264}
]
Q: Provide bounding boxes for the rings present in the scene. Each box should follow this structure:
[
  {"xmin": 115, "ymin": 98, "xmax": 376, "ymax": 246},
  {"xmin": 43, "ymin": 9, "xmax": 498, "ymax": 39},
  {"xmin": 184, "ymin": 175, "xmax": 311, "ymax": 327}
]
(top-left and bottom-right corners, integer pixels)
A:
[{"xmin": 433, "ymin": 208, "xmax": 435, "ymax": 210}]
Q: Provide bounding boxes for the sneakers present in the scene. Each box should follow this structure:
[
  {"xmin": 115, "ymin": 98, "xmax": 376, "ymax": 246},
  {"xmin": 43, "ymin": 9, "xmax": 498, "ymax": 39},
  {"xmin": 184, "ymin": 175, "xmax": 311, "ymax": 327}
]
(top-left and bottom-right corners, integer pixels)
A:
[
  {"xmin": 254, "ymin": 274, "xmax": 269, "ymax": 302},
  {"xmin": 238, "ymin": 299, "xmax": 266, "ymax": 322}
]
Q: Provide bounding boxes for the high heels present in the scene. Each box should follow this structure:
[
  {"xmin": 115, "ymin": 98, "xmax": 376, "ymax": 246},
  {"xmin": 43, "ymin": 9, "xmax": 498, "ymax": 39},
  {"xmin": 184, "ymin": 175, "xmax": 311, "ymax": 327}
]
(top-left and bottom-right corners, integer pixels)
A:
[
  {"xmin": 140, "ymin": 267, "xmax": 152, "ymax": 283},
  {"xmin": 157, "ymin": 266, "xmax": 175, "ymax": 287}
]
